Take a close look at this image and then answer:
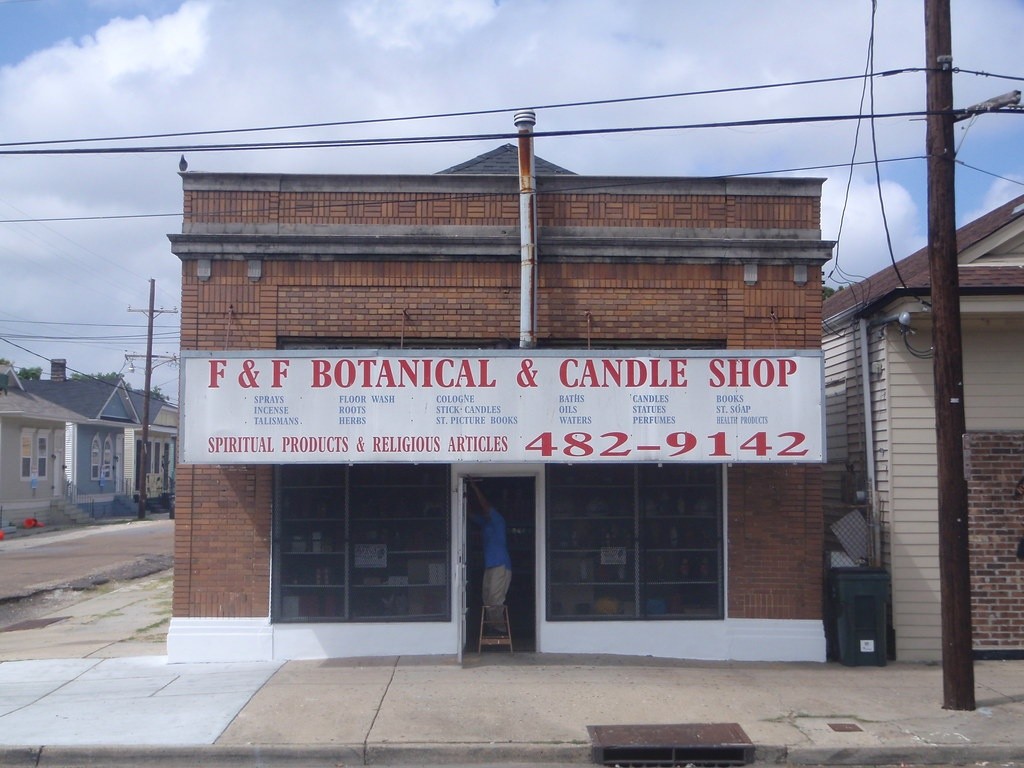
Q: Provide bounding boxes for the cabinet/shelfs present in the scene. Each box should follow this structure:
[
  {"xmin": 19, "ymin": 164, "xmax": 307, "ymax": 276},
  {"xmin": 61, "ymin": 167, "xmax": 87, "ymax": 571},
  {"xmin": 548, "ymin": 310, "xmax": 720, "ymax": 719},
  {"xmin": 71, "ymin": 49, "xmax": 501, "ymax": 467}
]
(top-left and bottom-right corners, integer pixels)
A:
[
  {"xmin": 279, "ymin": 464, "xmax": 451, "ymax": 621},
  {"xmin": 545, "ymin": 463, "xmax": 724, "ymax": 620}
]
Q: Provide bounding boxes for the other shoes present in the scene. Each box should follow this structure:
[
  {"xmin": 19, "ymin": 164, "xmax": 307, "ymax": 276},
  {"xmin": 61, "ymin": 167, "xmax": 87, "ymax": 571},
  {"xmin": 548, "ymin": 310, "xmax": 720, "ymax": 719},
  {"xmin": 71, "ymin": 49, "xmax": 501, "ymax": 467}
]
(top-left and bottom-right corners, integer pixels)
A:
[{"xmin": 483, "ymin": 629, "xmax": 507, "ymax": 637}]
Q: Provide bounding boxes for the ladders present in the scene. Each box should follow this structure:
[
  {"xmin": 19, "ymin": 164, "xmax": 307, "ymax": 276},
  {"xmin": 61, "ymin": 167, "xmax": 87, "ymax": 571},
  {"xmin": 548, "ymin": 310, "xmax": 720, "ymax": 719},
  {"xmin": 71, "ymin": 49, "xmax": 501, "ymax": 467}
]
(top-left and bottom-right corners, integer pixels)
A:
[{"xmin": 475, "ymin": 603, "xmax": 514, "ymax": 654}]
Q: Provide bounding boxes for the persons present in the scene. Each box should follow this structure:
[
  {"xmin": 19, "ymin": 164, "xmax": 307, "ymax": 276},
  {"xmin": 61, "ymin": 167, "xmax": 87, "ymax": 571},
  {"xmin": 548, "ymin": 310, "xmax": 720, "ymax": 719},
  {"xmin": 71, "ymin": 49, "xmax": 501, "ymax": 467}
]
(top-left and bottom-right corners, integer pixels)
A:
[{"xmin": 461, "ymin": 474, "xmax": 512, "ymax": 632}]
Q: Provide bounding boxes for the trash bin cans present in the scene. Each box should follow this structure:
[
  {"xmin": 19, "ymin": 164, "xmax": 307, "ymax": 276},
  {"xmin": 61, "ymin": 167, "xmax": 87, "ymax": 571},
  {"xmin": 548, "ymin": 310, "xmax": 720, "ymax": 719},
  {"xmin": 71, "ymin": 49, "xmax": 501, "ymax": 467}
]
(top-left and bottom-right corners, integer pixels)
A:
[{"xmin": 828, "ymin": 565, "xmax": 891, "ymax": 667}]
